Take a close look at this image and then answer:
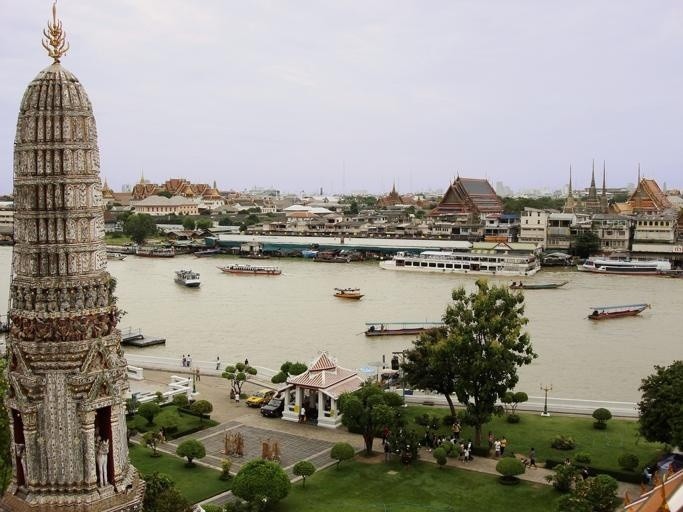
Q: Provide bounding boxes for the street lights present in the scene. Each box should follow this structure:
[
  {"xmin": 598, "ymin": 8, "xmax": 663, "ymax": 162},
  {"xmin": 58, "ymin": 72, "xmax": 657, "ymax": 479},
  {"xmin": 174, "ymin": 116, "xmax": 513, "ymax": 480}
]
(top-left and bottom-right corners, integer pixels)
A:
[
  {"xmin": 537, "ymin": 381, "xmax": 554, "ymax": 418},
  {"xmin": 391, "ymin": 348, "xmax": 414, "ymax": 409}
]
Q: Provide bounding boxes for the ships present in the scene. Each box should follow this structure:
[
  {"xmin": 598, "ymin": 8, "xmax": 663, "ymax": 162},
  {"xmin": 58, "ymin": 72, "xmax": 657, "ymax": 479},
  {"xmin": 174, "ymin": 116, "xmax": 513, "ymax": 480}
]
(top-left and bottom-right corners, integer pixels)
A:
[{"xmin": 377, "ymin": 248, "xmax": 541, "ymax": 278}]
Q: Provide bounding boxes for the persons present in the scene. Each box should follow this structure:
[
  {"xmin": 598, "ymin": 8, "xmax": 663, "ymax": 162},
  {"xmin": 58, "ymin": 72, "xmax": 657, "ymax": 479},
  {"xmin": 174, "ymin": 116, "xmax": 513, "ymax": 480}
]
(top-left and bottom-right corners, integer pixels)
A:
[
  {"xmin": 245, "ymin": 358, "xmax": 249, "ymax": 366},
  {"xmin": 643, "ymin": 466, "xmax": 653, "ymax": 483},
  {"xmin": 289, "ymin": 394, "xmax": 329, "ymax": 424},
  {"xmin": 564, "ymin": 457, "xmax": 570, "ymax": 467},
  {"xmin": 235, "ymin": 392, "xmax": 240, "ymax": 406},
  {"xmin": 216, "ymin": 357, "xmax": 221, "ymax": 370},
  {"xmin": 195, "ymin": 368, "xmax": 201, "ymax": 381},
  {"xmin": 158, "ymin": 426, "xmax": 168, "ymax": 443},
  {"xmin": 582, "ymin": 467, "xmax": 589, "ymax": 479},
  {"xmin": 182, "ymin": 354, "xmax": 187, "ymax": 367},
  {"xmin": 9, "ymin": 284, "xmax": 111, "ymax": 341},
  {"xmin": 382, "ymin": 421, "xmax": 507, "ymax": 463},
  {"xmin": 230, "ymin": 389, "xmax": 235, "ymax": 403},
  {"xmin": 187, "ymin": 354, "xmax": 192, "ymax": 366},
  {"xmin": 527, "ymin": 448, "xmax": 537, "ymax": 469}
]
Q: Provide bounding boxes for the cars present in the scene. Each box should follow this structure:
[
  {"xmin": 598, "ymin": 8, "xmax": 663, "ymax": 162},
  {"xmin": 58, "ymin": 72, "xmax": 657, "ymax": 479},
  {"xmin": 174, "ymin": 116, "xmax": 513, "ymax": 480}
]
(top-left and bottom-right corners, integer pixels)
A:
[
  {"xmin": 244, "ymin": 387, "xmax": 304, "ymax": 419},
  {"xmin": 642, "ymin": 465, "xmax": 656, "ymax": 485}
]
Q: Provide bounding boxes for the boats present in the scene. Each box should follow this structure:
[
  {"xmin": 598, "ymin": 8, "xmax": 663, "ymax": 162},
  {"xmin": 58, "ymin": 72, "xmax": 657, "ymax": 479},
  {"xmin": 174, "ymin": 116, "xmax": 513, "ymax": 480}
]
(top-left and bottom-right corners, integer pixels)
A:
[
  {"xmin": 193, "ymin": 248, "xmax": 222, "ymax": 257},
  {"xmin": 507, "ymin": 279, "xmax": 568, "ymax": 290},
  {"xmin": 0, "ymin": 313, "xmax": 8, "ymax": 334},
  {"xmin": 216, "ymin": 264, "xmax": 282, "ymax": 276},
  {"xmin": 542, "ymin": 252, "xmax": 573, "ymax": 268},
  {"xmin": 173, "ymin": 269, "xmax": 200, "ymax": 289},
  {"xmin": 575, "ymin": 256, "xmax": 672, "ymax": 276},
  {"xmin": 133, "ymin": 245, "xmax": 176, "ymax": 257},
  {"xmin": 586, "ymin": 302, "xmax": 651, "ymax": 320},
  {"xmin": 238, "ymin": 252, "xmax": 269, "ymax": 259},
  {"xmin": 106, "ymin": 251, "xmax": 127, "ymax": 261},
  {"xmin": 312, "ymin": 256, "xmax": 345, "ymax": 263},
  {"xmin": 332, "ymin": 287, "xmax": 363, "ymax": 301},
  {"xmin": 359, "ymin": 319, "xmax": 462, "ymax": 336}
]
